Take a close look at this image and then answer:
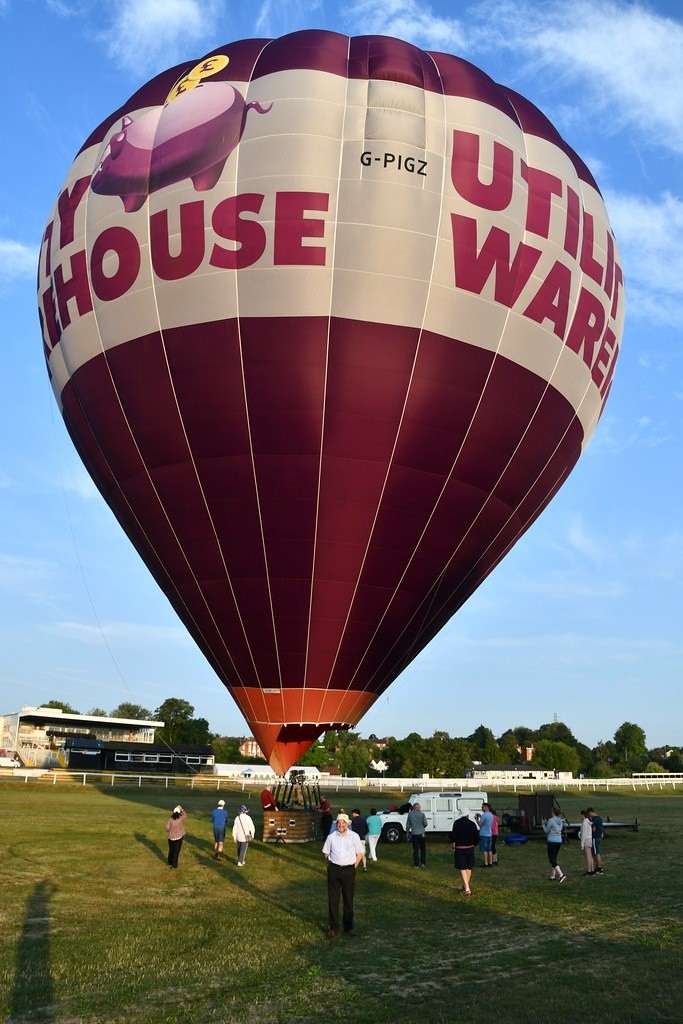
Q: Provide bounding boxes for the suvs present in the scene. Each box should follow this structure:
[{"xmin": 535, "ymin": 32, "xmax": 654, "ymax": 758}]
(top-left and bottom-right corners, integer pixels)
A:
[
  {"xmin": 0, "ymin": 757, "xmax": 21, "ymax": 768},
  {"xmin": 373, "ymin": 791, "xmax": 489, "ymax": 843}
]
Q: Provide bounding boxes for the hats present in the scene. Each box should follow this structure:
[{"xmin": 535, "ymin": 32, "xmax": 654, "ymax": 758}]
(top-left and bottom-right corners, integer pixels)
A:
[
  {"xmin": 332, "ymin": 813, "xmax": 352, "ymax": 826},
  {"xmin": 173, "ymin": 807, "xmax": 180, "ymax": 813},
  {"xmin": 458, "ymin": 806, "xmax": 471, "ymax": 816},
  {"xmin": 237, "ymin": 805, "xmax": 249, "ymax": 814},
  {"xmin": 217, "ymin": 800, "xmax": 225, "ymax": 806}
]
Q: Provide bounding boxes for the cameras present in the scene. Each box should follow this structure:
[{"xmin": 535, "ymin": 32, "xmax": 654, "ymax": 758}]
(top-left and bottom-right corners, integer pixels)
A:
[{"xmin": 475, "ymin": 814, "xmax": 480, "ymax": 819}]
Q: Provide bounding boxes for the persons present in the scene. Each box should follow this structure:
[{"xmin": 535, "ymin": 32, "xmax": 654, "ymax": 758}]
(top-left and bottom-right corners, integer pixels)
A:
[
  {"xmin": 366, "ymin": 808, "xmax": 383, "ymax": 861},
  {"xmin": 350, "ymin": 809, "xmax": 369, "ymax": 871},
  {"xmin": 330, "ymin": 809, "xmax": 351, "ymax": 833},
  {"xmin": 542, "ymin": 808, "xmax": 566, "ymax": 883},
  {"xmin": 282, "ymin": 788, "xmax": 305, "ymax": 809},
  {"xmin": 315, "ymin": 793, "xmax": 333, "ymax": 841},
  {"xmin": 581, "ymin": 810, "xmax": 595, "ymax": 876},
  {"xmin": 165, "ymin": 805, "xmax": 187, "ymax": 870},
  {"xmin": 212, "ymin": 800, "xmax": 228, "ymax": 860},
  {"xmin": 261, "ymin": 785, "xmax": 280, "ymax": 811},
  {"xmin": 588, "ymin": 807, "xmax": 603, "ymax": 874},
  {"xmin": 475, "ymin": 803, "xmax": 498, "ymax": 867},
  {"xmin": 406, "ymin": 803, "xmax": 428, "ymax": 868},
  {"xmin": 322, "ymin": 814, "xmax": 363, "ymax": 937},
  {"xmin": 451, "ymin": 807, "xmax": 480, "ymax": 896},
  {"xmin": 232, "ymin": 805, "xmax": 255, "ymax": 866}
]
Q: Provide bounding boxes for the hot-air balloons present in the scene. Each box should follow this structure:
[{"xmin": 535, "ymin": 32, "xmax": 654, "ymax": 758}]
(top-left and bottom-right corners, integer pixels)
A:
[{"xmin": 37, "ymin": 30, "xmax": 625, "ymax": 843}]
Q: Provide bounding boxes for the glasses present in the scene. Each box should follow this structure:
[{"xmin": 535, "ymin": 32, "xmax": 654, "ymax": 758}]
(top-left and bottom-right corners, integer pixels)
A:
[{"xmin": 482, "ymin": 806, "xmax": 485, "ymax": 808}]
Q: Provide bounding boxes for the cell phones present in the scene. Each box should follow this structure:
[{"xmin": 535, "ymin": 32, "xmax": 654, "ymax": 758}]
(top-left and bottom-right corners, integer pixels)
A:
[{"xmin": 542, "ymin": 816, "xmax": 545, "ymax": 820}]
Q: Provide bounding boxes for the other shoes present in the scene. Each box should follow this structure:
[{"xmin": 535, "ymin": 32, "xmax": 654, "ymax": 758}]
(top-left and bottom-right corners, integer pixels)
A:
[
  {"xmin": 467, "ymin": 892, "xmax": 472, "ymax": 897},
  {"xmin": 351, "ymin": 808, "xmax": 360, "ymax": 814},
  {"xmin": 215, "ymin": 851, "xmax": 223, "ymax": 861},
  {"xmin": 328, "ymin": 929, "xmax": 337, "ymax": 937},
  {"xmin": 593, "ymin": 867, "xmax": 604, "ymax": 874},
  {"xmin": 363, "ymin": 867, "xmax": 367, "ymax": 872},
  {"xmin": 369, "ymin": 857, "xmax": 377, "ymax": 861},
  {"xmin": 558, "ymin": 874, "xmax": 566, "ymax": 883},
  {"xmin": 480, "ymin": 863, "xmax": 488, "ymax": 868},
  {"xmin": 548, "ymin": 876, "xmax": 556, "ymax": 881},
  {"xmin": 344, "ymin": 929, "xmax": 354, "ymax": 936},
  {"xmin": 421, "ymin": 864, "xmax": 426, "ymax": 868},
  {"xmin": 237, "ymin": 861, "xmax": 245, "ymax": 866},
  {"xmin": 413, "ymin": 865, "xmax": 419, "ymax": 869},
  {"xmin": 492, "ymin": 861, "xmax": 498, "ymax": 866},
  {"xmin": 582, "ymin": 870, "xmax": 595, "ymax": 877},
  {"xmin": 488, "ymin": 862, "xmax": 493, "ymax": 868},
  {"xmin": 462, "ymin": 885, "xmax": 465, "ymax": 890}
]
